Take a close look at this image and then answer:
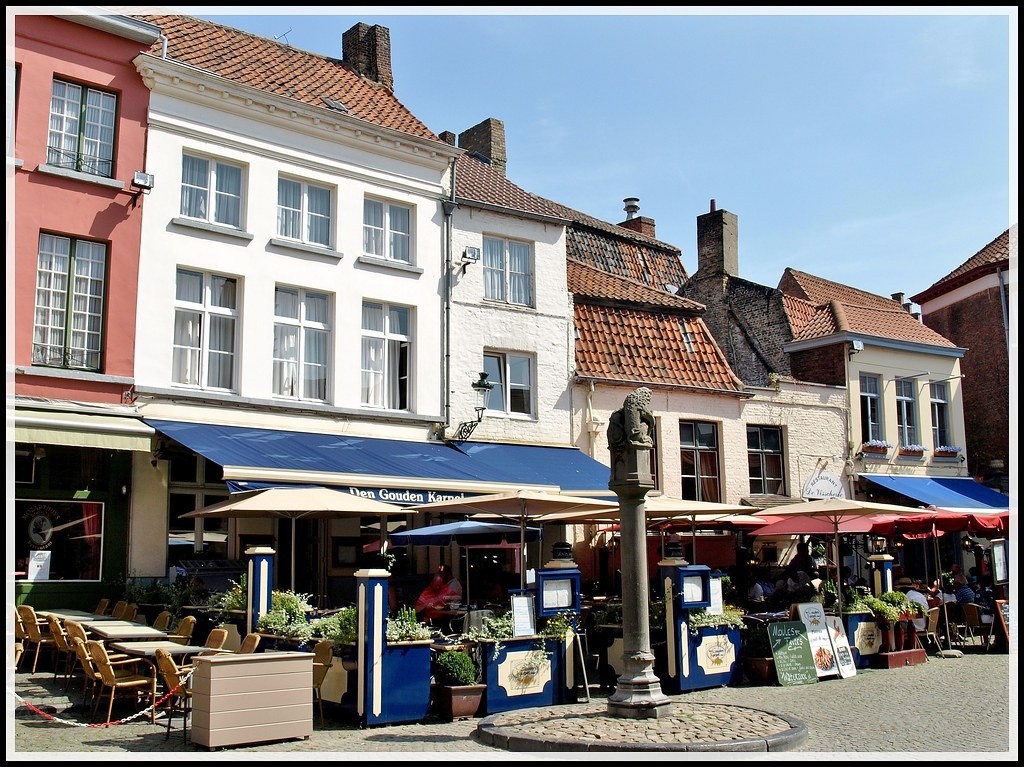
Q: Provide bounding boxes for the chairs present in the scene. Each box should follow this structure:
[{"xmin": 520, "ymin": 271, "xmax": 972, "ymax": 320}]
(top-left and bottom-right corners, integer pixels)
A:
[
  {"xmin": 235, "ymin": 633, "xmax": 260, "ymax": 653},
  {"xmin": 167, "ymin": 616, "xmax": 196, "ymax": 666},
  {"xmin": 155, "ymin": 649, "xmax": 192, "ymax": 745},
  {"xmin": 46, "ymin": 614, "xmax": 91, "ymax": 688},
  {"xmin": 199, "ymin": 628, "xmax": 228, "ymax": 656},
  {"xmin": 62, "ymin": 619, "xmax": 90, "ymax": 696},
  {"xmin": 93, "ymin": 599, "xmax": 109, "ymax": 615},
  {"xmin": 18, "ymin": 605, "xmax": 55, "ymax": 676},
  {"xmin": 150, "ymin": 611, "xmax": 172, "ymax": 632},
  {"xmin": 914, "ymin": 601, "xmax": 992, "ymax": 661},
  {"xmin": 312, "ymin": 640, "xmax": 334, "ymax": 731},
  {"xmin": 119, "ymin": 605, "xmax": 137, "ymax": 620},
  {"xmin": 15, "ymin": 605, "xmax": 29, "ymax": 671},
  {"xmin": 112, "ymin": 601, "xmax": 127, "ymax": 619},
  {"xmin": 72, "ymin": 637, "xmax": 133, "ymax": 715},
  {"xmin": 85, "ymin": 639, "xmax": 155, "ymax": 726}
]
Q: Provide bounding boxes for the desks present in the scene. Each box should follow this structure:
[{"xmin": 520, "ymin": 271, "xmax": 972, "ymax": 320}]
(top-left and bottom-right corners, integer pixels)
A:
[
  {"xmin": 190, "ymin": 652, "xmax": 318, "ymax": 756},
  {"xmin": 35, "ymin": 609, "xmax": 234, "ymax": 718}
]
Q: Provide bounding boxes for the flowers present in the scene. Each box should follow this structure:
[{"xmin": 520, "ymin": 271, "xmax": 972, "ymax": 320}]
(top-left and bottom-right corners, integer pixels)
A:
[
  {"xmin": 813, "ymin": 579, "xmax": 918, "ymax": 620},
  {"xmin": 198, "ymin": 573, "xmax": 248, "ymax": 627},
  {"xmin": 935, "ymin": 446, "xmax": 963, "ymax": 453},
  {"xmin": 432, "ymin": 611, "xmax": 513, "ymax": 661},
  {"xmin": 535, "ymin": 610, "xmax": 576, "ymax": 665},
  {"xmin": 689, "ymin": 601, "xmax": 748, "ymax": 639},
  {"xmin": 900, "ymin": 443, "xmax": 929, "ymax": 452},
  {"xmin": 115, "ymin": 571, "xmax": 195, "ymax": 616},
  {"xmin": 865, "ymin": 439, "xmax": 893, "ymax": 449},
  {"xmin": 258, "ymin": 590, "xmax": 432, "ymax": 648}
]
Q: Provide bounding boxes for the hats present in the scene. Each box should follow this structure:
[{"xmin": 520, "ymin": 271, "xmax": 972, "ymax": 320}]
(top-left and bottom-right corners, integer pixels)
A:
[{"xmin": 895, "ymin": 578, "xmax": 914, "ymax": 588}]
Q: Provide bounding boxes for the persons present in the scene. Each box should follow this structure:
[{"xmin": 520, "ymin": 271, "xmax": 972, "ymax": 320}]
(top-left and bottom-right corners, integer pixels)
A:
[
  {"xmin": 894, "ymin": 563, "xmax": 989, "ymax": 641},
  {"xmin": 736, "ymin": 542, "xmax": 867, "ymax": 602},
  {"xmin": 414, "ymin": 574, "xmax": 461, "ymax": 626},
  {"xmin": 438, "ymin": 564, "xmax": 462, "ymax": 608},
  {"xmin": 389, "ymin": 580, "xmax": 399, "ymax": 620}
]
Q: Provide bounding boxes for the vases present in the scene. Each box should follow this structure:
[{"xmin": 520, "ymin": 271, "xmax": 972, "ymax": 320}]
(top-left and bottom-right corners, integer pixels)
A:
[
  {"xmin": 876, "ymin": 617, "xmax": 896, "ymax": 653},
  {"xmin": 893, "ymin": 622, "xmax": 904, "ymax": 651},
  {"xmin": 746, "ymin": 657, "xmax": 773, "ymax": 686},
  {"xmin": 935, "ymin": 451, "xmax": 957, "ymax": 458},
  {"xmin": 432, "ymin": 635, "xmax": 476, "ymax": 659},
  {"xmin": 899, "ymin": 448, "xmax": 923, "ymax": 456},
  {"xmin": 862, "ymin": 444, "xmax": 888, "ymax": 454}
]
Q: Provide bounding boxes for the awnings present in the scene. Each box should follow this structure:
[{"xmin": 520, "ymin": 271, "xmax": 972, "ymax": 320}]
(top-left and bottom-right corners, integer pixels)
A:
[
  {"xmin": 141, "ymin": 416, "xmax": 620, "ymax": 505},
  {"xmin": 15, "ymin": 409, "xmax": 155, "ymax": 452},
  {"xmin": 859, "ymin": 473, "xmax": 1009, "ymax": 510}
]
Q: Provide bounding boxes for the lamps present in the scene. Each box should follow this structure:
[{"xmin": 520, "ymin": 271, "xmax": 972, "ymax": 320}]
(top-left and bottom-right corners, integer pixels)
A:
[
  {"xmin": 126, "ymin": 171, "xmax": 156, "ymax": 209},
  {"xmin": 848, "ymin": 340, "xmax": 865, "ymax": 362},
  {"xmin": 451, "ymin": 369, "xmax": 494, "ymax": 439},
  {"xmin": 461, "ymin": 245, "xmax": 480, "ymax": 274}
]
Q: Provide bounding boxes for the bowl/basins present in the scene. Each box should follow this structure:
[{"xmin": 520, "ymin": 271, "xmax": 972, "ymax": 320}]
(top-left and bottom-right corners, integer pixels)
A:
[
  {"xmin": 448, "ymin": 603, "xmax": 461, "ymax": 609},
  {"xmin": 432, "ymin": 606, "xmax": 444, "ymax": 611}
]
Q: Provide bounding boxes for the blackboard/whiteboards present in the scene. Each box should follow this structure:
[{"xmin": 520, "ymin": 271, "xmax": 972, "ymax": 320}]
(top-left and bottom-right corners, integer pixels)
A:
[
  {"xmin": 766, "ymin": 620, "xmax": 818, "ymax": 687},
  {"xmin": 995, "ymin": 600, "xmax": 1009, "ymax": 641}
]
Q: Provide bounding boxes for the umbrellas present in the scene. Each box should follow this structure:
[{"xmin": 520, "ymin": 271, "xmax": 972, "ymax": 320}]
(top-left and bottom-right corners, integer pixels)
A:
[
  {"xmin": 401, "ymin": 487, "xmax": 620, "ymax": 598},
  {"xmin": 868, "ymin": 505, "xmax": 1004, "ymax": 649},
  {"xmin": 986, "ymin": 510, "xmax": 1008, "ymax": 541},
  {"xmin": 178, "ymin": 486, "xmax": 419, "ymax": 594},
  {"xmin": 388, "ymin": 515, "xmax": 543, "ymax": 606},
  {"xmin": 868, "ymin": 504, "xmax": 1007, "ymax": 652},
  {"xmin": 532, "ymin": 494, "xmax": 772, "ymax": 620},
  {"xmin": 646, "ymin": 515, "xmax": 766, "ymax": 567},
  {"xmin": 746, "ymin": 496, "xmax": 938, "ymax": 626},
  {"xmin": 595, "ymin": 516, "xmax": 794, "ymax": 566},
  {"xmin": 747, "ymin": 509, "xmax": 906, "ymax": 616}
]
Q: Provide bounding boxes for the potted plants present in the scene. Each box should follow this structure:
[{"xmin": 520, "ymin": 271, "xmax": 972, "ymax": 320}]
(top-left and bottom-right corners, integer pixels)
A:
[{"xmin": 431, "ymin": 651, "xmax": 488, "ymax": 722}]
